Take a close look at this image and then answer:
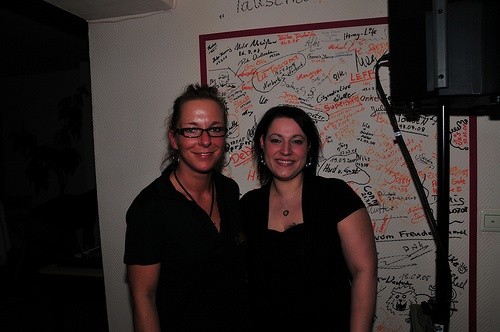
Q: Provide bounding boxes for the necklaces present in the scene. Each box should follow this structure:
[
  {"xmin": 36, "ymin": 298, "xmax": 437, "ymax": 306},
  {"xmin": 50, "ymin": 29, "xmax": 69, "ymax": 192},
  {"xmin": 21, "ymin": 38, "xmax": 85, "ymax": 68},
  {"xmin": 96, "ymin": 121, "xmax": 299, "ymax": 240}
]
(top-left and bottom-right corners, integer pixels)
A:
[
  {"xmin": 173, "ymin": 167, "xmax": 214, "ymax": 217},
  {"xmin": 275, "ymin": 189, "xmax": 289, "ymax": 217}
]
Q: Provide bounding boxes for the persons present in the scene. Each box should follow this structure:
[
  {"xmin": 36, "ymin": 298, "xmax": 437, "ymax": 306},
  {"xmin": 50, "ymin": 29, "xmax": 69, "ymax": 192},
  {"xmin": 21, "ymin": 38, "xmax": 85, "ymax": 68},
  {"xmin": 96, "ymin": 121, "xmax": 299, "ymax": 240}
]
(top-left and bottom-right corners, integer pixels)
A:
[
  {"xmin": 239, "ymin": 106, "xmax": 378, "ymax": 332},
  {"xmin": 123, "ymin": 81, "xmax": 240, "ymax": 332}
]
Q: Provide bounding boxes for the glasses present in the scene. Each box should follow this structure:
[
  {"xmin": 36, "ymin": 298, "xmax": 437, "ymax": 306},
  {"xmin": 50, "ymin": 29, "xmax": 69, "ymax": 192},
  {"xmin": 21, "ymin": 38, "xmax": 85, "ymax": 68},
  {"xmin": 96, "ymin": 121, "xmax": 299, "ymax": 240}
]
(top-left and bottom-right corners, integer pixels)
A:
[{"xmin": 174, "ymin": 127, "xmax": 227, "ymax": 138}]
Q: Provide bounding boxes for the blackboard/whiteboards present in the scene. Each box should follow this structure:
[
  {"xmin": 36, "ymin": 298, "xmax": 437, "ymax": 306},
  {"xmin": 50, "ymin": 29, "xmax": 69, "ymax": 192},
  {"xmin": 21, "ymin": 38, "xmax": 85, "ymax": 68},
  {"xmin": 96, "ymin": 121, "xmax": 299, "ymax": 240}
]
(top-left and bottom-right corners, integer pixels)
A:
[{"xmin": 198, "ymin": 17, "xmax": 476, "ymax": 332}]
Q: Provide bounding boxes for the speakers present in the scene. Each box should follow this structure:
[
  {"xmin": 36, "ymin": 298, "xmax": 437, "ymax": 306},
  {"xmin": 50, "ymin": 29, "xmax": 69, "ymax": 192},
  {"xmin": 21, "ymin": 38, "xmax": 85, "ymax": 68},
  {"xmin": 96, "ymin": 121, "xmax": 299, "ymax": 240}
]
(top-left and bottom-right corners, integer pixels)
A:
[{"xmin": 387, "ymin": 0, "xmax": 500, "ymax": 121}]
